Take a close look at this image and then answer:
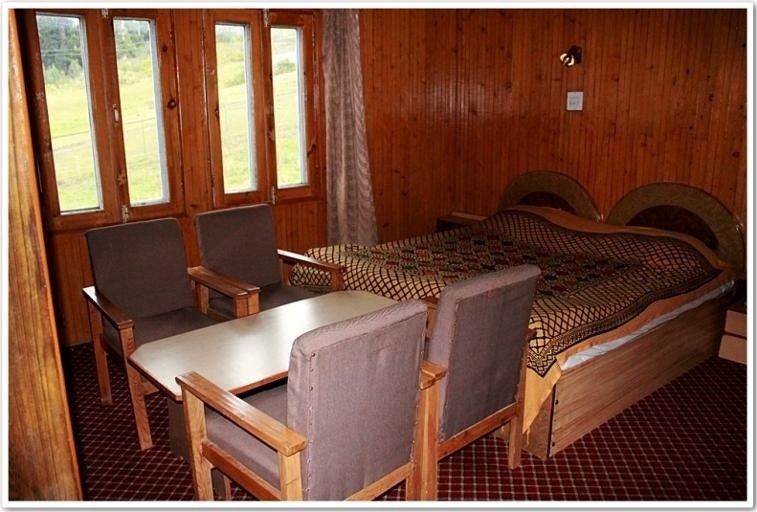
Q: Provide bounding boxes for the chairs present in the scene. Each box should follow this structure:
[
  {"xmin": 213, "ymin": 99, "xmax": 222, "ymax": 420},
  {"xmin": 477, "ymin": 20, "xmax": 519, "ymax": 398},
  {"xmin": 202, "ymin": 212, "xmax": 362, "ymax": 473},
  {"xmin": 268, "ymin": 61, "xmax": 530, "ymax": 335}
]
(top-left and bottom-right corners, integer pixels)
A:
[
  {"xmin": 191, "ymin": 203, "xmax": 343, "ymax": 316},
  {"xmin": 417, "ymin": 262, "xmax": 542, "ymax": 507},
  {"xmin": 173, "ymin": 298, "xmax": 433, "ymax": 505},
  {"xmin": 79, "ymin": 213, "xmax": 249, "ymax": 454}
]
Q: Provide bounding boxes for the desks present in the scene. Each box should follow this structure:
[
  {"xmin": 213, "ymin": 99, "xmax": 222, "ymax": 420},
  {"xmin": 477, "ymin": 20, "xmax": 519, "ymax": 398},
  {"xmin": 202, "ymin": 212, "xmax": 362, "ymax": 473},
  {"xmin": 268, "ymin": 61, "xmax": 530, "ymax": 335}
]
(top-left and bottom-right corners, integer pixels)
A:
[{"xmin": 126, "ymin": 289, "xmax": 402, "ymax": 501}]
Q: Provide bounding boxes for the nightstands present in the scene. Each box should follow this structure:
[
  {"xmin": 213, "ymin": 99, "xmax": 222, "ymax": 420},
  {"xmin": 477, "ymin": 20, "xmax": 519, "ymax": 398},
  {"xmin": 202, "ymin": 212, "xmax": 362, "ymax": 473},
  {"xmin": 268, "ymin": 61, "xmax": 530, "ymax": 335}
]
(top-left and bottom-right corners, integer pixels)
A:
[{"xmin": 718, "ymin": 297, "xmax": 745, "ymax": 366}]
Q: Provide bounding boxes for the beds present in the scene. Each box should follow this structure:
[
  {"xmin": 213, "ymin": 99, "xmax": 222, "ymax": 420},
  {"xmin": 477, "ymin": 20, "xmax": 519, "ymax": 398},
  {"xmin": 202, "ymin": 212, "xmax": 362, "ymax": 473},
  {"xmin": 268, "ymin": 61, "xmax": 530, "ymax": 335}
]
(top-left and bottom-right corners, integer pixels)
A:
[{"xmin": 288, "ymin": 170, "xmax": 744, "ymax": 466}]
